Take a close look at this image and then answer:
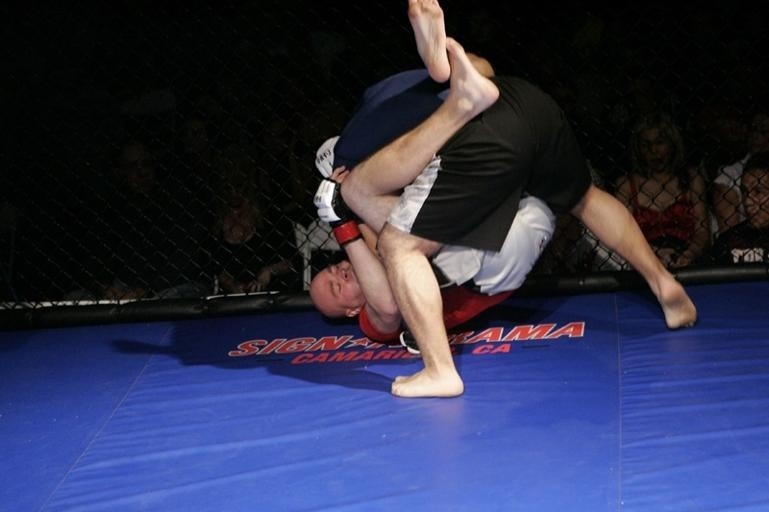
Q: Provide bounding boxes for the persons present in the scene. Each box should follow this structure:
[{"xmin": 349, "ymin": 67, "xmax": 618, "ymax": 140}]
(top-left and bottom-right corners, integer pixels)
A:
[
  {"xmin": 332, "ymin": 68, "xmax": 696, "ymax": 398},
  {"xmin": 309, "ymin": 1, "xmax": 559, "ymax": 346},
  {"xmin": 101, "ymin": 129, "xmax": 322, "ymax": 298},
  {"xmin": 531, "ymin": 117, "xmax": 768, "ymax": 276}
]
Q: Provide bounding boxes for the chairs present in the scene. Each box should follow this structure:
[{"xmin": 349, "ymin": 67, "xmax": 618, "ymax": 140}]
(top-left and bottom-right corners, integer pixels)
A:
[
  {"xmin": 304, "ymin": 217, "xmax": 351, "ymax": 294},
  {"xmin": 210, "ymin": 220, "xmax": 307, "ymax": 298}
]
